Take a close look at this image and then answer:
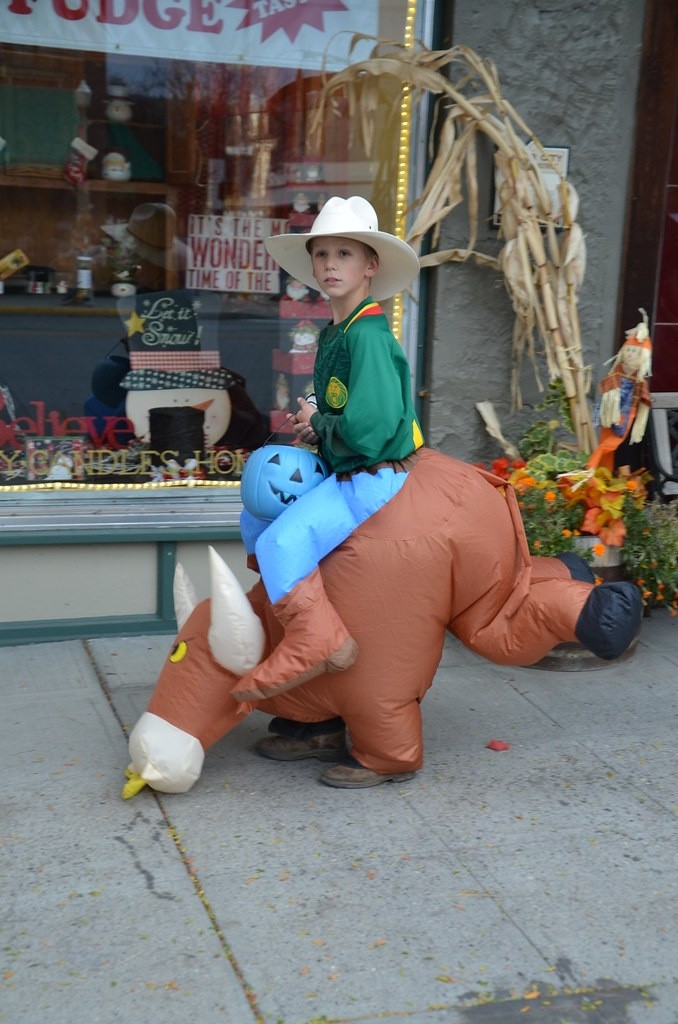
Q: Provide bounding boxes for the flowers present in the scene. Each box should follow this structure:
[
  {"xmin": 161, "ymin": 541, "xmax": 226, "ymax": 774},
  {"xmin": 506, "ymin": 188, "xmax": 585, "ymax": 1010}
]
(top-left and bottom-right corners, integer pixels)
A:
[{"xmin": 474, "ymin": 376, "xmax": 678, "ymax": 617}]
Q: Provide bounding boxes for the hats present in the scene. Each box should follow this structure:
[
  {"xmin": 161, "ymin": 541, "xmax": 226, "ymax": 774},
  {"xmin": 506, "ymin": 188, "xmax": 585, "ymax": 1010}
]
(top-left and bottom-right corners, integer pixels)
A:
[
  {"xmin": 265, "ymin": 196, "xmax": 420, "ymax": 303},
  {"xmin": 100, "ymin": 203, "xmax": 194, "ymax": 271}
]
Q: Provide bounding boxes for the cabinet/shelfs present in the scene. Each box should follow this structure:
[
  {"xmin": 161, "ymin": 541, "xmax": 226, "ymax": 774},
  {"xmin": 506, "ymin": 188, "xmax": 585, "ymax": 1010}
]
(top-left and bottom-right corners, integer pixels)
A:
[{"xmin": 0, "ymin": 41, "xmax": 224, "ymax": 440}]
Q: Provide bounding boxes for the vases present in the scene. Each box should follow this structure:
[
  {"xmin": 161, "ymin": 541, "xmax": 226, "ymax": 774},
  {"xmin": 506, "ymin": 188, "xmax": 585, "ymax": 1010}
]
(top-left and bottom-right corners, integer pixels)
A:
[{"xmin": 519, "ymin": 534, "xmax": 643, "ymax": 671}]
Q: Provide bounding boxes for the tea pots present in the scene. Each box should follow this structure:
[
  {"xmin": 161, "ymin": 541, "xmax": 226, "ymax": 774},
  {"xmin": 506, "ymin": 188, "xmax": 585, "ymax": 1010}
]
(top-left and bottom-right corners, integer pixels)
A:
[
  {"xmin": 101, "ymin": 153, "xmax": 132, "ymax": 181},
  {"xmin": 102, "ymin": 98, "xmax": 136, "ymax": 122}
]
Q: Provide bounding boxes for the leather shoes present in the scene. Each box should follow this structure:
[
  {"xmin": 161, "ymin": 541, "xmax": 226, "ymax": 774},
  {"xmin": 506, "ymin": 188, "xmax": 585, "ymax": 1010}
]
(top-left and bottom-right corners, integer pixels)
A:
[
  {"xmin": 318, "ymin": 758, "xmax": 416, "ymax": 789},
  {"xmin": 255, "ymin": 728, "xmax": 345, "ymax": 762}
]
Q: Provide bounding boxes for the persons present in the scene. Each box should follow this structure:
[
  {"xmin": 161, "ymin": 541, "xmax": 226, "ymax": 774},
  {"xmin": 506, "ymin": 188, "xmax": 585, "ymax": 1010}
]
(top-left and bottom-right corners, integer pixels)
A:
[
  {"xmin": 101, "ymin": 205, "xmax": 201, "ymax": 352},
  {"xmin": 264, "ymin": 197, "xmax": 426, "ymax": 788}
]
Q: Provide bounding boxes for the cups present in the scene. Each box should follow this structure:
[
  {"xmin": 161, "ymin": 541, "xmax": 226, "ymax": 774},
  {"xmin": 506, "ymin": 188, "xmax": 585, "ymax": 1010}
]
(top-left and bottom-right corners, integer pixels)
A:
[{"xmin": 112, "ymin": 283, "xmax": 137, "ymax": 298}]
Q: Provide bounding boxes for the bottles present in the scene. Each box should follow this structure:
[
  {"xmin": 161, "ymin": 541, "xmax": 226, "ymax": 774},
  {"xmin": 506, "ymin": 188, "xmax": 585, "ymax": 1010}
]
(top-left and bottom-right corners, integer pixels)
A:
[
  {"xmin": 23, "ymin": 256, "xmax": 95, "ymax": 300},
  {"xmin": 74, "ymin": 80, "xmax": 91, "ymax": 124}
]
